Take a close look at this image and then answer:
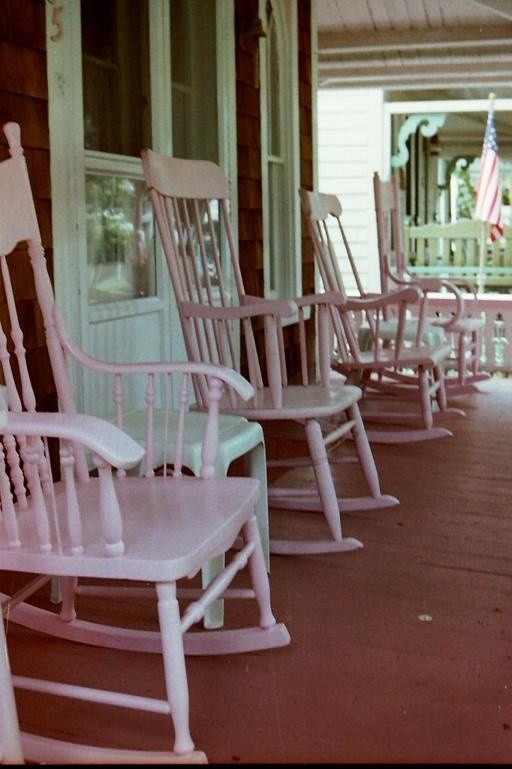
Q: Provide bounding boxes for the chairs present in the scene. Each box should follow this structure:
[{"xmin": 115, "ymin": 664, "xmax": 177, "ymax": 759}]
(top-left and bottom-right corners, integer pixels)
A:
[
  {"xmin": 491, "ymin": 223, "xmax": 512, "ymax": 267},
  {"xmin": 132, "ymin": 145, "xmax": 403, "ymax": 557},
  {"xmin": 298, "ymin": 182, "xmax": 467, "ymax": 445},
  {"xmin": 0, "ymin": 121, "xmax": 293, "ymax": 753},
  {"xmin": 370, "ymin": 162, "xmax": 494, "ymax": 396}
]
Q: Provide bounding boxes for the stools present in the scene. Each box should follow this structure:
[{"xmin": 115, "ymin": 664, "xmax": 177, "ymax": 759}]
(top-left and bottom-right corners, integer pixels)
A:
[
  {"xmin": 53, "ymin": 401, "xmax": 272, "ymax": 632},
  {"xmin": 347, "ymin": 312, "xmax": 449, "ymax": 412}
]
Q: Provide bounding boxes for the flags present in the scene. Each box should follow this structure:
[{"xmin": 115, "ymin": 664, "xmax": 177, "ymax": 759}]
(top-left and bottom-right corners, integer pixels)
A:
[{"xmin": 473, "ymin": 93, "xmax": 504, "ymax": 245}]
